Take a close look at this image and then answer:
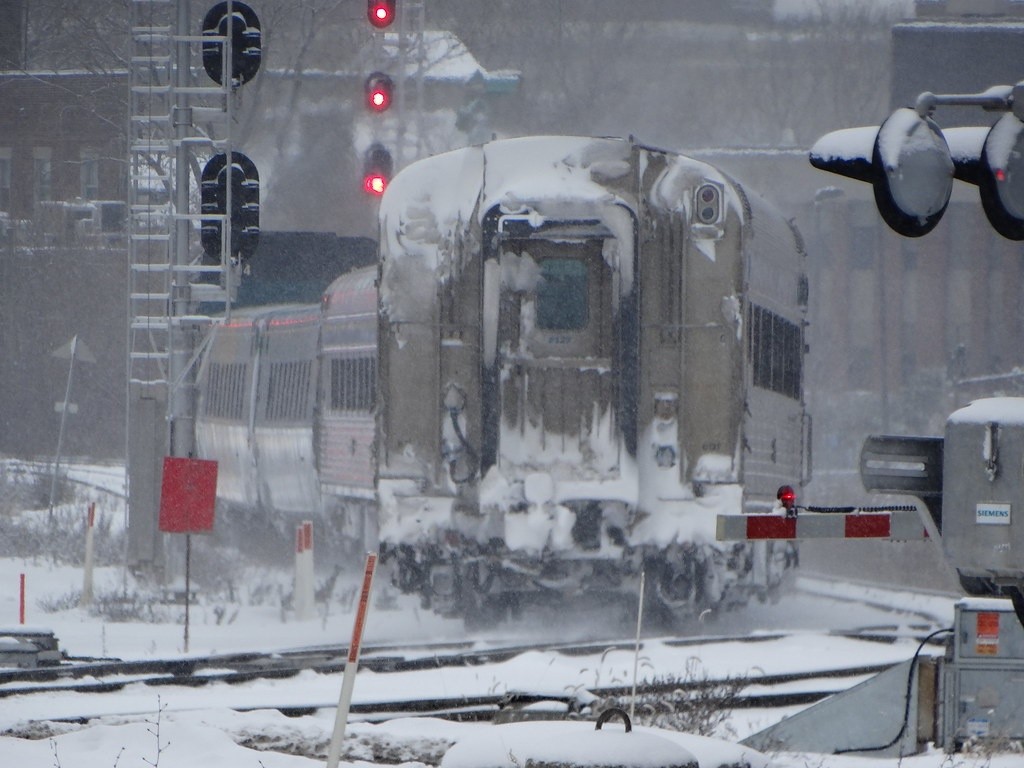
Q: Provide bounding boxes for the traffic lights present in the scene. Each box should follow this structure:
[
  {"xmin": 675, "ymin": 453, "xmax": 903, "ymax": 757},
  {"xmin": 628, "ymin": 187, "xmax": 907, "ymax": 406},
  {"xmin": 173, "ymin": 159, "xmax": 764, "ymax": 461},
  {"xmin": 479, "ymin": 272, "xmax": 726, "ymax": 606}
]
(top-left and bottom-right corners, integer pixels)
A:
[
  {"xmin": 363, "ymin": 72, "xmax": 394, "ymax": 115},
  {"xmin": 363, "ymin": 144, "xmax": 395, "ymax": 196},
  {"xmin": 368, "ymin": 0, "xmax": 397, "ymax": 29}
]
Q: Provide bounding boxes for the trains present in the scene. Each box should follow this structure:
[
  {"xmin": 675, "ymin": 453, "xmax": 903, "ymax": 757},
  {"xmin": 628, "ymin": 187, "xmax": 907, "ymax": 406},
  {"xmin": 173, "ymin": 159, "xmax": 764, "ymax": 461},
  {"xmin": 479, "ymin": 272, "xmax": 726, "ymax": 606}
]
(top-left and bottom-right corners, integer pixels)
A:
[{"xmin": 162, "ymin": 132, "xmax": 819, "ymax": 633}]
